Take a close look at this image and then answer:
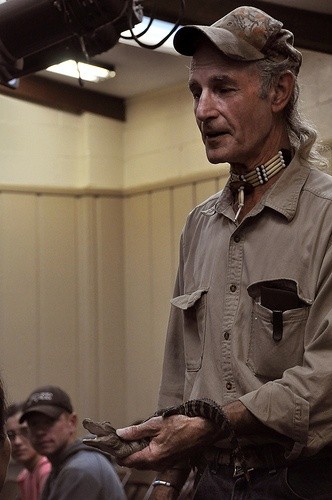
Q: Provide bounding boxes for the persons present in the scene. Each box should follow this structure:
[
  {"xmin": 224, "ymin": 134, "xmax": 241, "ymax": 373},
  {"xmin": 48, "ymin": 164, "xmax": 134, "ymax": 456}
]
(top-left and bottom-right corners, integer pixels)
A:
[
  {"xmin": 6, "ymin": 404, "xmax": 52, "ymax": 500},
  {"xmin": 115, "ymin": 6, "xmax": 332, "ymax": 500},
  {"xmin": 19, "ymin": 385, "xmax": 129, "ymax": 500}
]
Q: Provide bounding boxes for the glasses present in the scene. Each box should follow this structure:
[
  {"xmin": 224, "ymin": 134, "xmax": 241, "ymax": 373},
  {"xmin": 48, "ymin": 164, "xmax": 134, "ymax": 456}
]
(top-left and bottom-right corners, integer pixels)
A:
[{"xmin": 7, "ymin": 430, "xmax": 31, "ymax": 442}]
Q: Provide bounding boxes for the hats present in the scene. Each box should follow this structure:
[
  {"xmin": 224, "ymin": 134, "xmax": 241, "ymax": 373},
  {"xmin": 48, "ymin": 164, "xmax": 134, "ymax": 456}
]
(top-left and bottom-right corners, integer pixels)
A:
[
  {"xmin": 18, "ymin": 385, "xmax": 74, "ymax": 424},
  {"xmin": 172, "ymin": 6, "xmax": 303, "ymax": 76}
]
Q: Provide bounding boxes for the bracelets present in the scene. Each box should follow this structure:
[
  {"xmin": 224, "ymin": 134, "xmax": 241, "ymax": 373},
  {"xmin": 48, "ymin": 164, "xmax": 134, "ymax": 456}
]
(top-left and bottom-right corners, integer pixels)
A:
[{"xmin": 151, "ymin": 479, "xmax": 180, "ymax": 495}]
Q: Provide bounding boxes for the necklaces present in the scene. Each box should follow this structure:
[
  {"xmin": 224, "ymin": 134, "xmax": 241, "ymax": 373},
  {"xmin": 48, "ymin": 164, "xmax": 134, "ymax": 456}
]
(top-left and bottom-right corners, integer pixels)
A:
[{"xmin": 227, "ymin": 146, "xmax": 297, "ymax": 225}]
